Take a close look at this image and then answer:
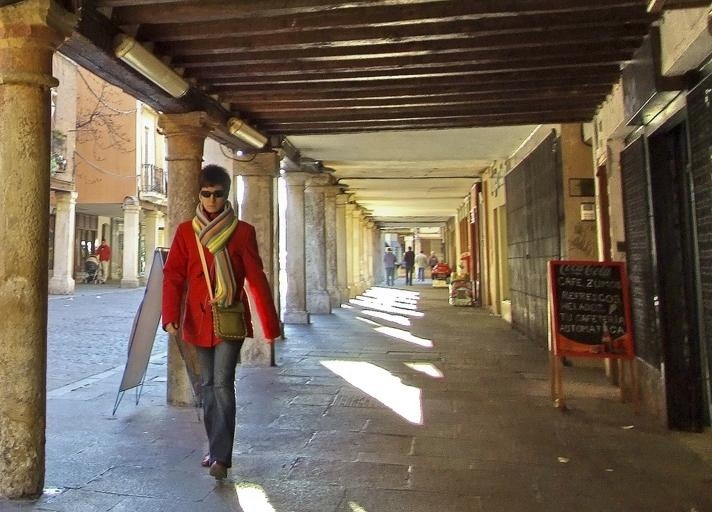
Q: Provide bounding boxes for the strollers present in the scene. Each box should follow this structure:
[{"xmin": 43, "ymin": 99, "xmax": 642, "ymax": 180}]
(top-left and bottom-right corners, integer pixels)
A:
[{"xmin": 83, "ymin": 254, "xmax": 102, "ymax": 284}]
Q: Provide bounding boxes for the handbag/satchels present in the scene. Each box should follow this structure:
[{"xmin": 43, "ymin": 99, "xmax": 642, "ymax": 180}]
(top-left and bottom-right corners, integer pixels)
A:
[{"xmin": 211, "ymin": 301, "xmax": 249, "ymax": 339}]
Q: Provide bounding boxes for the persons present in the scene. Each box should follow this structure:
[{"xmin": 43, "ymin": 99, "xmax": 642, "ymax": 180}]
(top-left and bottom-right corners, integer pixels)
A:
[
  {"xmin": 95, "ymin": 238, "xmax": 112, "ymax": 283},
  {"xmin": 383, "ymin": 248, "xmax": 396, "ymax": 287},
  {"xmin": 153, "ymin": 161, "xmax": 284, "ymax": 483},
  {"xmin": 414, "ymin": 251, "xmax": 428, "ymax": 281},
  {"xmin": 426, "ymin": 251, "xmax": 439, "ymax": 281},
  {"xmin": 403, "ymin": 246, "xmax": 415, "ymax": 287}
]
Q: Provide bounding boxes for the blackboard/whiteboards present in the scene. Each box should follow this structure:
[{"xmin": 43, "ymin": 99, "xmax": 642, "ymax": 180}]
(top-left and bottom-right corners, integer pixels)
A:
[{"xmin": 547, "ymin": 259, "xmax": 635, "ymax": 360}]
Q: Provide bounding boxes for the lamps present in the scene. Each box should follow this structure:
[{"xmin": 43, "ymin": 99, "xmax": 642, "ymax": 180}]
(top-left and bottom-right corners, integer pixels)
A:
[
  {"xmin": 112, "ymin": 33, "xmax": 190, "ymax": 101},
  {"xmin": 224, "ymin": 118, "xmax": 268, "ymax": 150}
]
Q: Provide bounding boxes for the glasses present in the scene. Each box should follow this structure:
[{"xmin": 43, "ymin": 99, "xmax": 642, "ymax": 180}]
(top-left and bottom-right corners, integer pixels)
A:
[{"xmin": 200, "ymin": 190, "xmax": 226, "ymax": 198}]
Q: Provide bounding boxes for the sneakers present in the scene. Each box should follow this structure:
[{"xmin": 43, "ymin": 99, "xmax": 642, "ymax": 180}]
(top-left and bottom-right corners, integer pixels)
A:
[{"xmin": 202, "ymin": 451, "xmax": 231, "ymax": 480}]
